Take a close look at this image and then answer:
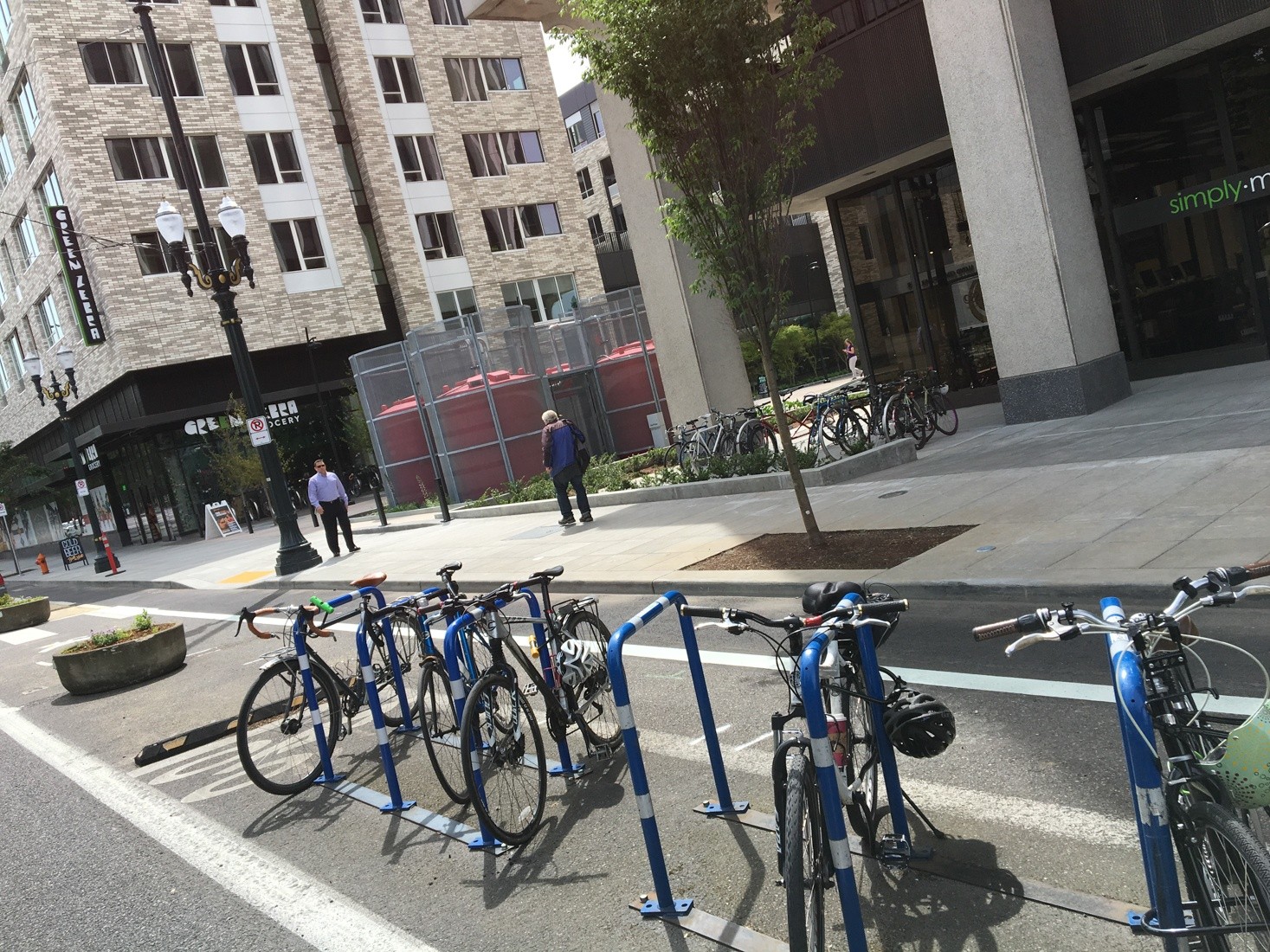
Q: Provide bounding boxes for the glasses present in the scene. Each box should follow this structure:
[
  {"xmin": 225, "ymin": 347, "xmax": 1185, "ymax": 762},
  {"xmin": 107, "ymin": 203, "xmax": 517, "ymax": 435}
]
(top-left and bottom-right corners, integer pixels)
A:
[{"xmin": 315, "ymin": 464, "xmax": 325, "ymax": 468}]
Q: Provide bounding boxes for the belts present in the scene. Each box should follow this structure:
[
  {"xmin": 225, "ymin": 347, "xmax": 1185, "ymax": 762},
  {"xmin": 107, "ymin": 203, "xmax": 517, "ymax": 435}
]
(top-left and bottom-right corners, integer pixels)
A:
[{"xmin": 319, "ymin": 497, "xmax": 338, "ymax": 504}]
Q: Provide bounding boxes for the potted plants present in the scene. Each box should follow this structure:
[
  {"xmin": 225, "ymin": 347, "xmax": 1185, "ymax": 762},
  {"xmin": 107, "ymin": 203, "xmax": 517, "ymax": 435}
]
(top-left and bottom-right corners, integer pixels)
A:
[
  {"xmin": 53, "ymin": 608, "xmax": 187, "ymax": 696},
  {"xmin": 0, "ymin": 593, "xmax": 51, "ymax": 634}
]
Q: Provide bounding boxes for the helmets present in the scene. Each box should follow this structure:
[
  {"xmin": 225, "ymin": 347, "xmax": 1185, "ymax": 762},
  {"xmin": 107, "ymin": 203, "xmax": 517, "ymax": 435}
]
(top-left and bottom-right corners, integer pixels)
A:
[
  {"xmin": 556, "ymin": 639, "xmax": 593, "ymax": 686},
  {"xmin": 882, "ymin": 687, "xmax": 956, "ymax": 758},
  {"xmin": 1200, "ymin": 699, "xmax": 1269, "ymax": 809}
]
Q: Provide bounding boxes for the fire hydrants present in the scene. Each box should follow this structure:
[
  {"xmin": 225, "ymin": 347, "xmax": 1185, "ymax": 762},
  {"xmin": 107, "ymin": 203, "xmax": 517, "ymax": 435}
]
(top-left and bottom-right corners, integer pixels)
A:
[{"xmin": 34, "ymin": 552, "xmax": 50, "ymax": 573}]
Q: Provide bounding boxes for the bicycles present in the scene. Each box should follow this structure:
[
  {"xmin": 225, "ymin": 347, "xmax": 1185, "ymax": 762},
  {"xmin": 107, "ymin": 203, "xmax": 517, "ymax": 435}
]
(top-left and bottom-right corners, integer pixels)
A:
[
  {"xmin": 679, "ymin": 577, "xmax": 946, "ymax": 952},
  {"xmin": 358, "ymin": 561, "xmax": 623, "ymax": 845},
  {"xmin": 968, "ymin": 559, "xmax": 1270, "ymax": 952},
  {"xmin": 662, "ymin": 365, "xmax": 959, "ymax": 481},
  {"xmin": 217, "ymin": 452, "xmax": 385, "ymax": 523},
  {"xmin": 233, "ymin": 569, "xmax": 430, "ymax": 796}
]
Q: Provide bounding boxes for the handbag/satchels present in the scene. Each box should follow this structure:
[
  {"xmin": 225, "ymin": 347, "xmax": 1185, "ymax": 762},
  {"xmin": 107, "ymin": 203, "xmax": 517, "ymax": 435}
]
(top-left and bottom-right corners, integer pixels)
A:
[{"xmin": 561, "ymin": 419, "xmax": 591, "ymax": 474}]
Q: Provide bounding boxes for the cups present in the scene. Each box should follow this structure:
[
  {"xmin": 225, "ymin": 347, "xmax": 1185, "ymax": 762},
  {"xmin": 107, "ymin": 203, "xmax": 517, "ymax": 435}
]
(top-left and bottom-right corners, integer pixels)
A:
[{"xmin": 827, "ymin": 713, "xmax": 850, "ymax": 767}]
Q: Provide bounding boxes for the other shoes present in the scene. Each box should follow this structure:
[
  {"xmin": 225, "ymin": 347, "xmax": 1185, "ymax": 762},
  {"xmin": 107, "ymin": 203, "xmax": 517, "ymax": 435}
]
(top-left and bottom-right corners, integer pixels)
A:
[
  {"xmin": 852, "ymin": 377, "xmax": 857, "ymax": 381},
  {"xmin": 349, "ymin": 546, "xmax": 361, "ymax": 552},
  {"xmin": 860, "ymin": 370, "xmax": 864, "ymax": 377},
  {"xmin": 558, "ymin": 514, "xmax": 577, "ymax": 526},
  {"xmin": 334, "ymin": 551, "xmax": 340, "ymax": 556},
  {"xmin": 579, "ymin": 511, "xmax": 593, "ymax": 522}
]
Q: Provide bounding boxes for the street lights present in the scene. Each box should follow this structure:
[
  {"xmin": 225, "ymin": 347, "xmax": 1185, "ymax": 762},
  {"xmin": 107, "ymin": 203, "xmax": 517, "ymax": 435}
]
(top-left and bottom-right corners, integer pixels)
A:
[
  {"xmin": 304, "ymin": 326, "xmax": 355, "ymax": 506},
  {"xmin": 125, "ymin": 3, "xmax": 324, "ymax": 578},
  {"xmin": 807, "ymin": 259, "xmax": 830, "ymax": 383},
  {"xmin": 22, "ymin": 344, "xmax": 122, "ymax": 574}
]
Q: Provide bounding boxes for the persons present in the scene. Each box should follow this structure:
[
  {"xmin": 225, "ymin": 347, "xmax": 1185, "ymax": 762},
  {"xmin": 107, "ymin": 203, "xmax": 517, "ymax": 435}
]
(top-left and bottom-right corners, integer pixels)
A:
[
  {"xmin": 843, "ymin": 339, "xmax": 864, "ymax": 380},
  {"xmin": 307, "ymin": 459, "xmax": 361, "ymax": 556},
  {"xmin": 541, "ymin": 409, "xmax": 594, "ymax": 524},
  {"xmin": 226, "ymin": 513, "xmax": 233, "ymax": 522}
]
